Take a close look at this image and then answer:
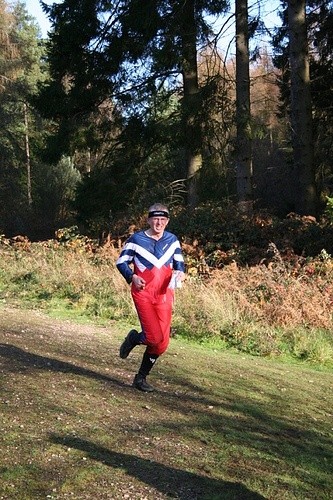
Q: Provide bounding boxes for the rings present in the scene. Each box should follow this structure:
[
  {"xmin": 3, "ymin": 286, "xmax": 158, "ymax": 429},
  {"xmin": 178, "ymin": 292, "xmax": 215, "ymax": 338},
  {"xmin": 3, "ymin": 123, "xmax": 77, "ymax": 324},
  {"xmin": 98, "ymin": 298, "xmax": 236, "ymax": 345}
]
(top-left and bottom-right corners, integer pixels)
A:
[{"xmin": 139, "ymin": 286, "xmax": 144, "ymax": 290}]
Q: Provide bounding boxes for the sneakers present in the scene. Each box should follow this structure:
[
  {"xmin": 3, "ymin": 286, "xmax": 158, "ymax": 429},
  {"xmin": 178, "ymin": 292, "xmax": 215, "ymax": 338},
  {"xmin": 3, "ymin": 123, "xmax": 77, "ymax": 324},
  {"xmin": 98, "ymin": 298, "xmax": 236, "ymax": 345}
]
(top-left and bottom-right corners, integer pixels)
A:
[
  {"xmin": 132, "ymin": 373, "xmax": 154, "ymax": 392},
  {"xmin": 119, "ymin": 329, "xmax": 139, "ymax": 359}
]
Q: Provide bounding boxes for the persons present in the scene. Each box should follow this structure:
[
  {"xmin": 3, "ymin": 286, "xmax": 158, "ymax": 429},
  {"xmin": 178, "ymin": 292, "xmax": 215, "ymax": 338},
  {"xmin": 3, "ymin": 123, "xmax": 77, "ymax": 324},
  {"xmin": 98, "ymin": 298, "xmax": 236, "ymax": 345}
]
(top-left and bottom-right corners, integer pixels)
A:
[{"xmin": 113, "ymin": 202, "xmax": 187, "ymax": 394}]
